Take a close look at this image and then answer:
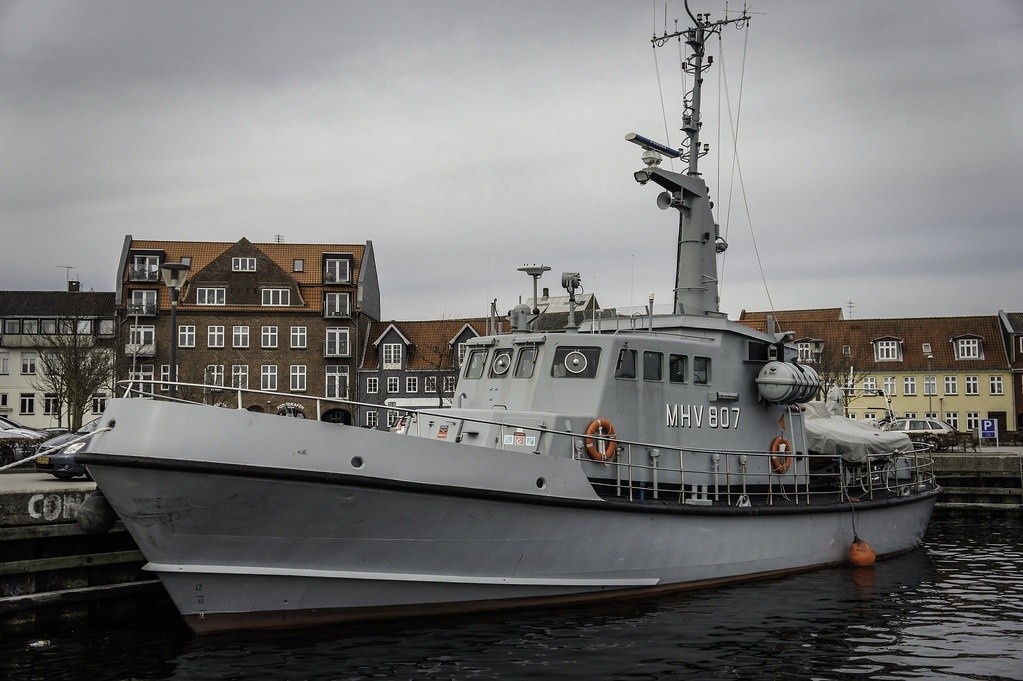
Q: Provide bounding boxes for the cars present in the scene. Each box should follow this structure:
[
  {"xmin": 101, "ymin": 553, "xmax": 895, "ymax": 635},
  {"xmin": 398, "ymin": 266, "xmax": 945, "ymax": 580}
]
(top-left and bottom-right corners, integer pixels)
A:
[
  {"xmin": 0, "ymin": 416, "xmax": 72, "ymax": 465},
  {"xmin": 34, "ymin": 414, "xmax": 102, "ymax": 481},
  {"xmin": 881, "ymin": 418, "xmax": 961, "ymax": 453}
]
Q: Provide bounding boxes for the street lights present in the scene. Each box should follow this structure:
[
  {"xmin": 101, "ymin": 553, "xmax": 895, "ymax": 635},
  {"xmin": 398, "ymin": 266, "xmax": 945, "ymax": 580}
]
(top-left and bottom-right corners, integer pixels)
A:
[
  {"xmin": 927, "ymin": 355, "xmax": 933, "ymax": 418},
  {"xmin": 160, "ymin": 261, "xmax": 190, "ymax": 401}
]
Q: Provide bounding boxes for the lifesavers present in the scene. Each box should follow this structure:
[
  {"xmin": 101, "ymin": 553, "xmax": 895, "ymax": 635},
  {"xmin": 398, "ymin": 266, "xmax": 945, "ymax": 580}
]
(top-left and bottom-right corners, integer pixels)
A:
[
  {"xmin": 771, "ymin": 436, "xmax": 791, "ymax": 472},
  {"xmin": 585, "ymin": 418, "xmax": 617, "ymax": 461}
]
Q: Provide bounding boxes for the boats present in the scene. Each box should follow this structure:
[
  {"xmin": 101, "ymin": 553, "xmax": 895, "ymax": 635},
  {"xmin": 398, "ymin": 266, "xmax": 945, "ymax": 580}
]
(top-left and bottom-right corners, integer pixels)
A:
[{"xmin": 61, "ymin": 0, "xmax": 944, "ymax": 629}]
